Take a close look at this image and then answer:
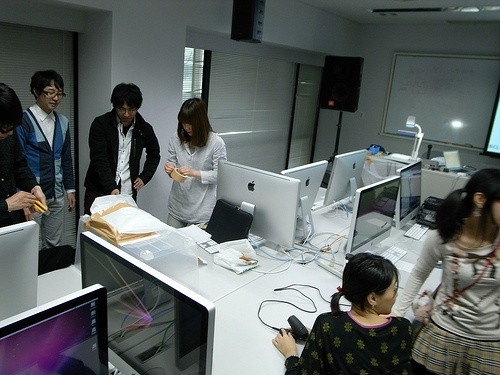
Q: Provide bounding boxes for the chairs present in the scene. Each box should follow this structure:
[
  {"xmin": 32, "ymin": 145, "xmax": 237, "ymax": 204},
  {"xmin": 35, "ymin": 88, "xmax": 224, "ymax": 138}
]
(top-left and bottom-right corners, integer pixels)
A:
[{"xmin": 420, "ymin": 150, "xmax": 445, "ymax": 160}]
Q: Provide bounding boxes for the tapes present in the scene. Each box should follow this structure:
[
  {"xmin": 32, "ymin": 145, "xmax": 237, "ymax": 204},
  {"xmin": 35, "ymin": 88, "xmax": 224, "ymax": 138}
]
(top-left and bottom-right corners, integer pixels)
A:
[{"xmin": 171, "ymin": 168, "xmax": 187, "ymax": 183}]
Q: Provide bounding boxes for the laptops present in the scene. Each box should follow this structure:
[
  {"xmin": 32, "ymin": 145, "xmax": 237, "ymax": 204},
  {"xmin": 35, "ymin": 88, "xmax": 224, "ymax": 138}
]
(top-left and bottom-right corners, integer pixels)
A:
[{"xmin": 443, "ymin": 151, "xmax": 472, "ymax": 171}]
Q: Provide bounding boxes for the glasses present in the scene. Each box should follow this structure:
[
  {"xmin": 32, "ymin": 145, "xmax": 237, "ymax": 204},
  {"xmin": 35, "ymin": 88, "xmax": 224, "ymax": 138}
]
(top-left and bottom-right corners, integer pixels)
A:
[
  {"xmin": 42, "ymin": 89, "xmax": 66, "ymax": 99},
  {"xmin": 0, "ymin": 123, "xmax": 21, "ymax": 134}
]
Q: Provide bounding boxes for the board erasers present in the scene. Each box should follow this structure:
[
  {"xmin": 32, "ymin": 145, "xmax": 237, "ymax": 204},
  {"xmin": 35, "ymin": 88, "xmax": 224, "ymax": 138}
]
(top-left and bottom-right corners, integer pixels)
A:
[{"xmin": 397, "ymin": 131, "xmax": 416, "ymax": 136}]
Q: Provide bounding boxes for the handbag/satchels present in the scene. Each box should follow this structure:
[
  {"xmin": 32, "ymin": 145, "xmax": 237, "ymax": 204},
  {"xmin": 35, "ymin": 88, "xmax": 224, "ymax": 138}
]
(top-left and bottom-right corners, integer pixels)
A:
[{"xmin": 410, "ymin": 290, "xmax": 434, "ymax": 325}]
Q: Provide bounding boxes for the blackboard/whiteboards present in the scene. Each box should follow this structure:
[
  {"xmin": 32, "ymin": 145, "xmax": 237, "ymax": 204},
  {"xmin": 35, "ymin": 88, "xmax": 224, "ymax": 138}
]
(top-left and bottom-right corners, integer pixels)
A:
[{"xmin": 380, "ymin": 51, "xmax": 500, "ymax": 152}]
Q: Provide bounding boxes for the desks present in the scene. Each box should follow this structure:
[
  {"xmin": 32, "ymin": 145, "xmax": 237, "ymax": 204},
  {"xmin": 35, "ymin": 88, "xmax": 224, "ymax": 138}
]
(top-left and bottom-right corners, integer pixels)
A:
[
  {"xmin": 35, "ymin": 175, "xmax": 446, "ymax": 375},
  {"xmin": 384, "ymin": 152, "xmax": 470, "ymax": 204}
]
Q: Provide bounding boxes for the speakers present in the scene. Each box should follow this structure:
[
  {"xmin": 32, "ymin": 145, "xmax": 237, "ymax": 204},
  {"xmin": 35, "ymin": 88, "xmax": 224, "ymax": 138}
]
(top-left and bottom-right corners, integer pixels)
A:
[
  {"xmin": 230, "ymin": 0, "xmax": 266, "ymax": 44},
  {"xmin": 318, "ymin": 55, "xmax": 365, "ymax": 114}
]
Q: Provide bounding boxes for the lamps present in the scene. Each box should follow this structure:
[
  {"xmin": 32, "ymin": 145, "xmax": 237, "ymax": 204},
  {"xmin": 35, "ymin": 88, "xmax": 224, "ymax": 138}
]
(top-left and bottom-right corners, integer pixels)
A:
[{"xmin": 405, "ymin": 115, "xmax": 422, "ymax": 160}]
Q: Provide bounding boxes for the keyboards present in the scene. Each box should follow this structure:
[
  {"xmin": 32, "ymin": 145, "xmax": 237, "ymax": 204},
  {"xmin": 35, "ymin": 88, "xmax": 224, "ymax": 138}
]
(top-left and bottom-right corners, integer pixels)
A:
[
  {"xmin": 310, "ymin": 198, "xmax": 323, "ymax": 212},
  {"xmin": 380, "ymin": 246, "xmax": 410, "ymax": 264},
  {"xmin": 247, "ymin": 230, "xmax": 264, "ymax": 249},
  {"xmin": 405, "ymin": 223, "xmax": 430, "ymax": 240}
]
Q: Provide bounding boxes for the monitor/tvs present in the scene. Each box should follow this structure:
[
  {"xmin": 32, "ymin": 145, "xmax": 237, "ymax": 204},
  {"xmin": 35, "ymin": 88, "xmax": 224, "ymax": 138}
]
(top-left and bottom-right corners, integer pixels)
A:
[
  {"xmin": 215, "ymin": 160, "xmax": 300, "ymax": 250},
  {"xmin": 1, "ymin": 218, "xmax": 40, "ymax": 322},
  {"xmin": 396, "ymin": 159, "xmax": 424, "ymax": 227},
  {"xmin": 79, "ymin": 231, "xmax": 216, "ymax": 375},
  {"xmin": 347, "ymin": 174, "xmax": 402, "ymax": 256},
  {"xmin": 325, "ymin": 148, "xmax": 368, "ymax": 210},
  {"xmin": 0, "ymin": 283, "xmax": 111, "ymax": 374},
  {"xmin": 280, "ymin": 160, "xmax": 328, "ymax": 242}
]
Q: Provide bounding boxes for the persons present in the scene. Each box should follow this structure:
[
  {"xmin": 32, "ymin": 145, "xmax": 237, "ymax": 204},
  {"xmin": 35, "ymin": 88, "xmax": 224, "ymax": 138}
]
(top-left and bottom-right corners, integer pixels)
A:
[
  {"xmin": 83, "ymin": 83, "xmax": 161, "ymax": 217},
  {"xmin": 272, "ymin": 252, "xmax": 413, "ymax": 375},
  {"xmin": 0, "ymin": 83, "xmax": 47, "ymax": 228},
  {"xmin": 392, "ymin": 168, "xmax": 500, "ymax": 375},
  {"xmin": 165, "ymin": 97, "xmax": 227, "ymax": 229},
  {"xmin": 16, "ymin": 70, "xmax": 76, "ymax": 251}
]
